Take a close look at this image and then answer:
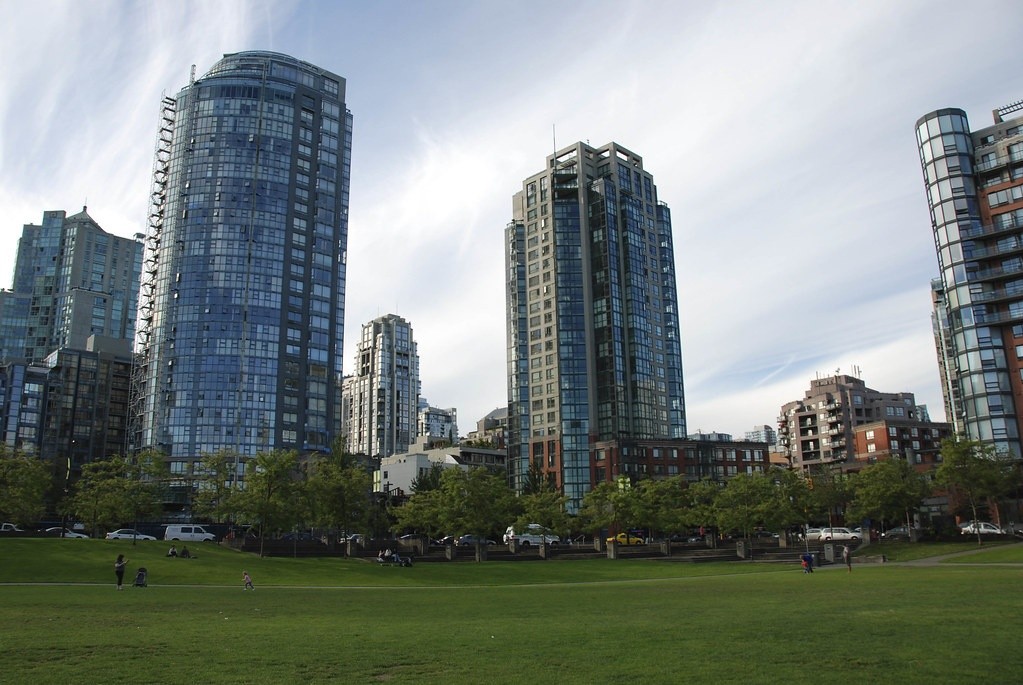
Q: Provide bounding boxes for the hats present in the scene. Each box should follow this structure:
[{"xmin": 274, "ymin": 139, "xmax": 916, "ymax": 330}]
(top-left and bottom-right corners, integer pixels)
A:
[{"xmin": 841, "ymin": 543, "xmax": 846, "ymax": 546}]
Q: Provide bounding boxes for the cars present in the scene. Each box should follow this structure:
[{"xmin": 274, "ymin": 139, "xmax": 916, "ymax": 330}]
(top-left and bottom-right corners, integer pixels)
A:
[
  {"xmin": 456, "ymin": 534, "xmax": 497, "ymax": 547},
  {"xmin": 105, "ymin": 528, "xmax": 157, "ymax": 541},
  {"xmin": 344, "ymin": 534, "xmax": 374, "ymax": 545},
  {"xmin": 687, "ymin": 535, "xmax": 707, "ymax": 543},
  {"xmin": 226, "ymin": 531, "xmax": 256, "ymax": 543},
  {"xmin": 770, "ymin": 525, "xmax": 919, "ymax": 544},
  {"xmin": 961, "ymin": 521, "xmax": 1007, "ymax": 536},
  {"xmin": 45, "ymin": 527, "xmax": 89, "ymax": 540},
  {"xmin": 438, "ymin": 536, "xmax": 455, "ymax": 546},
  {"xmin": 0, "ymin": 522, "xmax": 25, "ymax": 534},
  {"xmin": 607, "ymin": 533, "xmax": 644, "ymax": 546},
  {"xmin": 337, "ymin": 531, "xmax": 351, "ymax": 544},
  {"xmin": 279, "ymin": 532, "xmax": 326, "ymax": 546},
  {"xmin": 396, "ymin": 533, "xmax": 441, "ymax": 549}
]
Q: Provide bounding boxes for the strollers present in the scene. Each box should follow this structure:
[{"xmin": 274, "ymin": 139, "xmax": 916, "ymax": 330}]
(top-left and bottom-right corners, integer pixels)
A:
[{"xmin": 133, "ymin": 567, "xmax": 148, "ymax": 588}]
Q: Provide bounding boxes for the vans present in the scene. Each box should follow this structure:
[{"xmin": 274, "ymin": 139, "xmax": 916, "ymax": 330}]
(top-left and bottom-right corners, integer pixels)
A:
[{"xmin": 164, "ymin": 524, "xmax": 216, "ymax": 543}]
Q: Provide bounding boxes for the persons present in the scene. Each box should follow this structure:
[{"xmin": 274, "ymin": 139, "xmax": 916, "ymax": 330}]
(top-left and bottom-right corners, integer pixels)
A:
[
  {"xmin": 801, "ymin": 525, "xmax": 806, "ymax": 540},
  {"xmin": 872, "ymin": 528, "xmax": 877, "ymax": 539},
  {"xmin": 859, "ymin": 527, "xmax": 864, "ymax": 544},
  {"xmin": 115, "ymin": 554, "xmax": 128, "ymax": 590},
  {"xmin": 181, "ymin": 546, "xmax": 189, "ymax": 558},
  {"xmin": 378, "ymin": 548, "xmax": 401, "ymax": 562},
  {"xmin": 407, "ymin": 553, "xmax": 415, "ymax": 563},
  {"xmin": 972, "ymin": 523, "xmax": 975, "ymax": 533},
  {"xmin": 914, "ymin": 520, "xmax": 920, "ymax": 529},
  {"xmin": 455, "ymin": 540, "xmax": 459, "ymax": 546},
  {"xmin": 842, "ymin": 543, "xmax": 851, "ymax": 572},
  {"xmin": 169, "ymin": 545, "xmax": 177, "ymax": 558},
  {"xmin": 801, "ymin": 555, "xmax": 813, "ymax": 573},
  {"xmin": 241, "ymin": 571, "xmax": 255, "ymax": 591}
]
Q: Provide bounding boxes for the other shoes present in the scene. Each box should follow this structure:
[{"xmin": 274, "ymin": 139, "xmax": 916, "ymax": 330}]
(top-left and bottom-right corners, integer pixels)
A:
[
  {"xmin": 252, "ymin": 588, "xmax": 255, "ymax": 591},
  {"xmin": 243, "ymin": 588, "xmax": 247, "ymax": 591},
  {"xmin": 116, "ymin": 588, "xmax": 124, "ymax": 590}
]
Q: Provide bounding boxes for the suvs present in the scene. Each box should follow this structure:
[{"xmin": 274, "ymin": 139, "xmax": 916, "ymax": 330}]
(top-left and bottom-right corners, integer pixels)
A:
[{"xmin": 503, "ymin": 523, "xmax": 561, "ymax": 548}]
